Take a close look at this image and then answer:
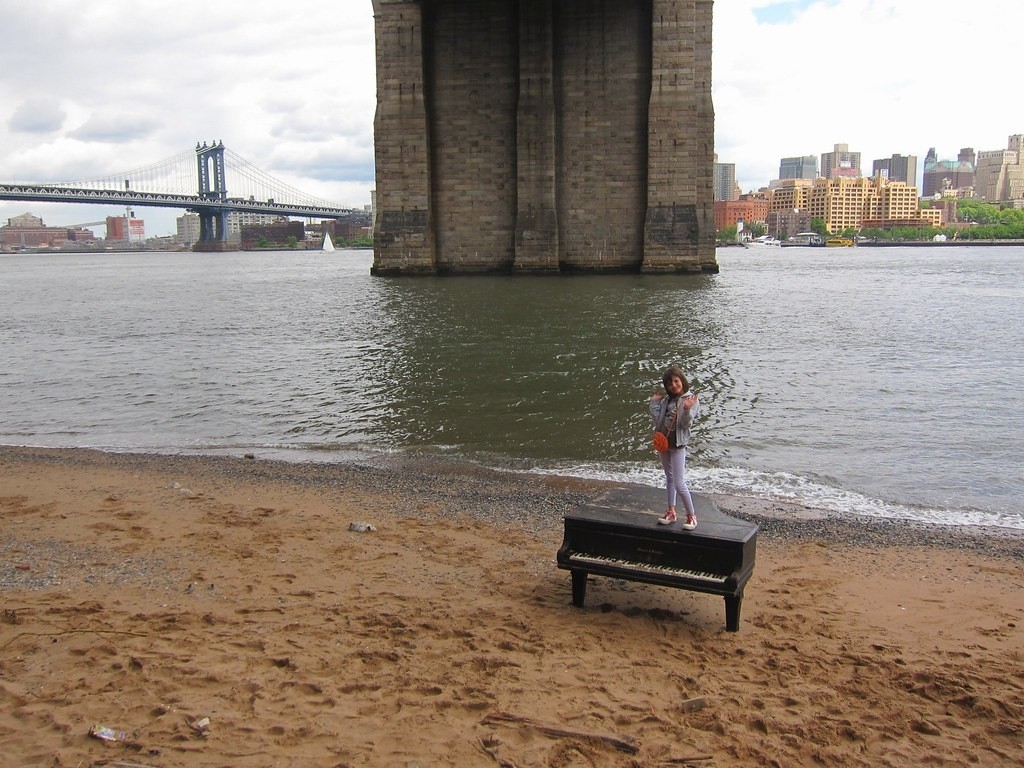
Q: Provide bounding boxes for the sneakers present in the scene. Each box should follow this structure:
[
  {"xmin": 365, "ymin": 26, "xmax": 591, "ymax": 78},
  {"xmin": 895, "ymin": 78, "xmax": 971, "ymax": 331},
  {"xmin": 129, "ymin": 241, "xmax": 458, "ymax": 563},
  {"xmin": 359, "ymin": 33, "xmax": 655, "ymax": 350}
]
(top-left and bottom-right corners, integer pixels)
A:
[
  {"xmin": 658, "ymin": 510, "xmax": 676, "ymax": 525},
  {"xmin": 682, "ymin": 514, "xmax": 697, "ymax": 530}
]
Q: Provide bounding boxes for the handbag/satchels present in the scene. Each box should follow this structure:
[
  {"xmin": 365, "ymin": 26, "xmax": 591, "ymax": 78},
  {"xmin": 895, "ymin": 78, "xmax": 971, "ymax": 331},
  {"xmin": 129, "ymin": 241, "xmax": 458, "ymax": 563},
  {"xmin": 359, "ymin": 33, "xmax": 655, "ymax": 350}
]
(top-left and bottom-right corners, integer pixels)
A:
[{"xmin": 653, "ymin": 432, "xmax": 668, "ymax": 451}]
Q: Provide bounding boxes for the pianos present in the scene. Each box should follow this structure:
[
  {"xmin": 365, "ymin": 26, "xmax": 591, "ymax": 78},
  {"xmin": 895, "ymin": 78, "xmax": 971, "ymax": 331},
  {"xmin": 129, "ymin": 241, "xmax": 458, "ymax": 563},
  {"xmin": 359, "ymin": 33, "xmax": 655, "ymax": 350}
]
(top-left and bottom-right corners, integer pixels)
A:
[{"xmin": 556, "ymin": 504, "xmax": 761, "ymax": 633}]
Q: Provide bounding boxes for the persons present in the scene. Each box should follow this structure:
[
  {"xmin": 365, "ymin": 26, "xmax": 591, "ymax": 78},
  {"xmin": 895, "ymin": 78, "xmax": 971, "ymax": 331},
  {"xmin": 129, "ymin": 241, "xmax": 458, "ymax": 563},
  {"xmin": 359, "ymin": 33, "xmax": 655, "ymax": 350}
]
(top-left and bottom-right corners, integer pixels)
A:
[{"xmin": 649, "ymin": 368, "xmax": 700, "ymax": 530}]
